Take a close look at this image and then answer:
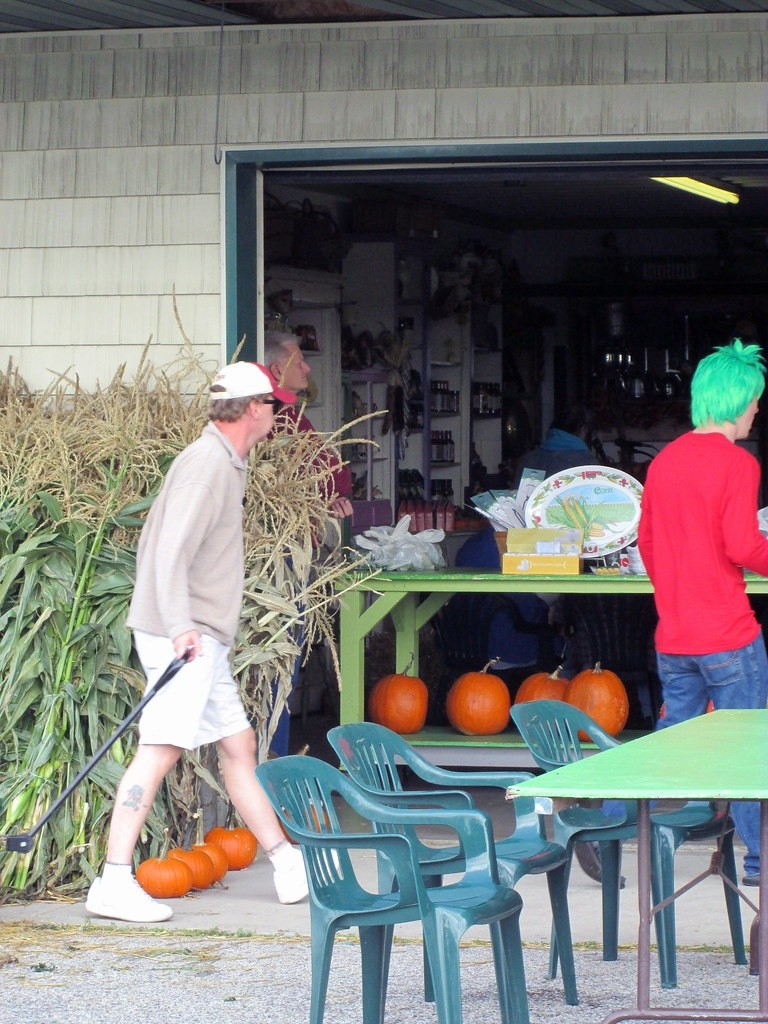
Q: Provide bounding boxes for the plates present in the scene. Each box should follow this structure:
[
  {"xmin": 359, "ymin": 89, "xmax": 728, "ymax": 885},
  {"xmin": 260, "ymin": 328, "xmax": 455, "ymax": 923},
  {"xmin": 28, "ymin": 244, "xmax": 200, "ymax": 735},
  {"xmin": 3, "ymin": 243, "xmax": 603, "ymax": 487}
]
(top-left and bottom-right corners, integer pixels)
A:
[{"xmin": 524, "ymin": 465, "xmax": 645, "ymax": 558}]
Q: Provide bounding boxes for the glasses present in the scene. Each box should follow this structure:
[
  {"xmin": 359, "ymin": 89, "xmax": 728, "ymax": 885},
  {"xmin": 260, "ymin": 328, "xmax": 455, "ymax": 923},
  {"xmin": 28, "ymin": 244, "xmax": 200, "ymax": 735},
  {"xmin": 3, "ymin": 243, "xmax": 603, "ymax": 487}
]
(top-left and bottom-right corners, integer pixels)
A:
[{"xmin": 260, "ymin": 399, "xmax": 281, "ymax": 414}]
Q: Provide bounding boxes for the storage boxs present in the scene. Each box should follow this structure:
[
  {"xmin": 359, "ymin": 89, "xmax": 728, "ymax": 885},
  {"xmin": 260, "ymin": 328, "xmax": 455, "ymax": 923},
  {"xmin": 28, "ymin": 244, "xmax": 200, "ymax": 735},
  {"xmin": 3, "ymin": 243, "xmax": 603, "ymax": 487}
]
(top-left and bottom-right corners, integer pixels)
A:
[{"xmin": 502, "ymin": 529, "xmax": 584, "ymax": 574}]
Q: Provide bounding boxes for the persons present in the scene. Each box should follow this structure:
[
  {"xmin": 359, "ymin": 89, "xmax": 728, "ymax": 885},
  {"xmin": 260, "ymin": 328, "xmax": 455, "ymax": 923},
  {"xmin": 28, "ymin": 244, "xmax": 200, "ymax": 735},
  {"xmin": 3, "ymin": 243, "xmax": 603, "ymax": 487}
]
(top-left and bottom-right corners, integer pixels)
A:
[
  {"xmin": 508, "ymin": 400, "xmax": 600, "ymax": 491},
  {"xmin": 262, "ymin": 328, "xmax": 353, "ymax": 758},
  {"xmin": 571, "ymin": 339, "xmax": 768, "ymax": 887},
  {"xmin": 83, "ymin": 361, "xmax": 341, "ymax": 925}
]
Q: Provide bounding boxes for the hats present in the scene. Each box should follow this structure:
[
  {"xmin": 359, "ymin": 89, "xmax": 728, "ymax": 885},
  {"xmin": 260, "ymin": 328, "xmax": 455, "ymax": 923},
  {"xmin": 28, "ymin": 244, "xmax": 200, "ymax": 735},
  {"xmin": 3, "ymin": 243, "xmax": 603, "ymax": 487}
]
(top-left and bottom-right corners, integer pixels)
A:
[
  {"xmin": 210, "ymin": 361, "xmax": 297, "ymax": 404},
  {"xmin": 549, "ymin": 401, "xmax": 589, "ymax": 429}
]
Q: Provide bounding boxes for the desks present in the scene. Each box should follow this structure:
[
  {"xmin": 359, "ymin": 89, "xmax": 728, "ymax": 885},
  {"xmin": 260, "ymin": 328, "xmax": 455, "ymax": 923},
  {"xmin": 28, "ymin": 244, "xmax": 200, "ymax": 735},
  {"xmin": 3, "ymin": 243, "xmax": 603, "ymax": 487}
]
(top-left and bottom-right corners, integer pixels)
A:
[{"xmin": 506, "ymin": 709, "xmax": 768, "ymax": 1024}]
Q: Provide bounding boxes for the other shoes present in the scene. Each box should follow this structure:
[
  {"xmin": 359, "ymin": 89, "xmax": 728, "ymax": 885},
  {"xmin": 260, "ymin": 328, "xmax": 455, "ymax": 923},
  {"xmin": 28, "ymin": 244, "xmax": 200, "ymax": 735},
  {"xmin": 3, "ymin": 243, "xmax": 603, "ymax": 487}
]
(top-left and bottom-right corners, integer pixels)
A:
[
  {"xmin": 574, "ymin": 839, "xmax": 624, "ymax": 888},
  {"xmin": 743, "ymin": 874, "xmax": 759, "ymax": 887}
]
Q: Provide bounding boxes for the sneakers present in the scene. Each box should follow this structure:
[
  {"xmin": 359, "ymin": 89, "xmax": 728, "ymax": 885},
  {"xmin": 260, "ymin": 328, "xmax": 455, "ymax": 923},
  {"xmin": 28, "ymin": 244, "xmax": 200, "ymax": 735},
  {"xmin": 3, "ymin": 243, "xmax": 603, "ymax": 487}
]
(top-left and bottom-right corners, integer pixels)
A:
[
  {"xmin": 85, "ymin": 877, "xmax": 173, "ymax": 922},
  {"xmin": 274, "ymin": 850, "xmax": 340, "ymax": 903}
]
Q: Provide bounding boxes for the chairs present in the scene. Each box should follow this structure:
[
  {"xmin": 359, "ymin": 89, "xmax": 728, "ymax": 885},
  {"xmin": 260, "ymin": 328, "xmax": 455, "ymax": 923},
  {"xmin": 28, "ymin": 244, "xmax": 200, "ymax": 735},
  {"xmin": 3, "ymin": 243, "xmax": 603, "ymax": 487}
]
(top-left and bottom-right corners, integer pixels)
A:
[
  {"xmin": 255, "ymin": 755, "xmax": 528, "ymax": 1024},
  {"xmin": 326, "ymin": 722, "xmax": 579, "ymax": 1024},
  {"xmin": 509, "ymin": 700, "xmax": 748, "ymax": 988}
]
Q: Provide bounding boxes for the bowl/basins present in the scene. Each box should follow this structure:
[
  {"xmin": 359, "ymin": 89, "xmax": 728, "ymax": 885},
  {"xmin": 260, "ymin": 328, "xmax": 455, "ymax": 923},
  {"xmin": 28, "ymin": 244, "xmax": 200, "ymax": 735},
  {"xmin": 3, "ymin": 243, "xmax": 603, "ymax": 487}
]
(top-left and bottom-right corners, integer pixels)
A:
[{"xmin": 627, "ymin": 547, "xmax": 647, "ymax": 575}]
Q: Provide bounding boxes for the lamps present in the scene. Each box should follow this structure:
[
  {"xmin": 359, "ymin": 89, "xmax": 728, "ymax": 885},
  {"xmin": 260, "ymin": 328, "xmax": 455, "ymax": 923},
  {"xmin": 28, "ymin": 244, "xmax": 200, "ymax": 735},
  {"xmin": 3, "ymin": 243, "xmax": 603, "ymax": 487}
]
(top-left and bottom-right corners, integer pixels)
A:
[{"xmin": 648, "ymin": 174, "xmax": 744, "ymax": 204}]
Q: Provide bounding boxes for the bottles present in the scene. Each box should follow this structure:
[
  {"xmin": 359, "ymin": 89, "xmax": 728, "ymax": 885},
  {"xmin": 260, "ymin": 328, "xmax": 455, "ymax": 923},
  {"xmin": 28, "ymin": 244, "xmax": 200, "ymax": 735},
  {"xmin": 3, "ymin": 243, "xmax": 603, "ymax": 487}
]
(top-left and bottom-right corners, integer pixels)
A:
[{"xmin": 428, "ymin": 379, "xmax": 504, "ymax": 506}]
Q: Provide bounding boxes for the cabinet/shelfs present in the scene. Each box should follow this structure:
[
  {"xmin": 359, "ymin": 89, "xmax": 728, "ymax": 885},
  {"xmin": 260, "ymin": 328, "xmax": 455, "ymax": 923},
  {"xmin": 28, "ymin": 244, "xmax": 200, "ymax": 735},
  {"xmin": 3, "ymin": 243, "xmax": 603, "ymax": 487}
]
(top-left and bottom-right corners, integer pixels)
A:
[
  {"xmin": 264, "ymin": 243, "xmax": 503, "ymax": 546},
  {"xmin": 334, "ymin": 567, "xmax": 768, "ymax": 770}
]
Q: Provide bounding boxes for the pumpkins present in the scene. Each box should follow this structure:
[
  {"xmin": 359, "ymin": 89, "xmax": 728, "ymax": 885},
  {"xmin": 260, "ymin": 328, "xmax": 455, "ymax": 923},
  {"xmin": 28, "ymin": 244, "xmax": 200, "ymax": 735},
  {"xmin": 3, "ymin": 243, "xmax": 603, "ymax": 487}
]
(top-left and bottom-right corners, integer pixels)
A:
[
  {"xmin": 369, "ymin": 653, "xmax": 428, "ymax": 734},
  {"xmin": 660, "ymin": 698, "xmax": 716, "ymax": 715},
  {"xmin": 136, "ymin": 826, "xmax": 257, "ymax": 899},
  {"xmin": 280, "ymin": 805, "xmax": 330, "ymax": 842},
  {"xmin": 446, "ymin": 660, "xmax": 511, "ymax": 735},
  {"xmin": 514, "ymin": 662, "xmax": 628, "ymax": 740}
]
